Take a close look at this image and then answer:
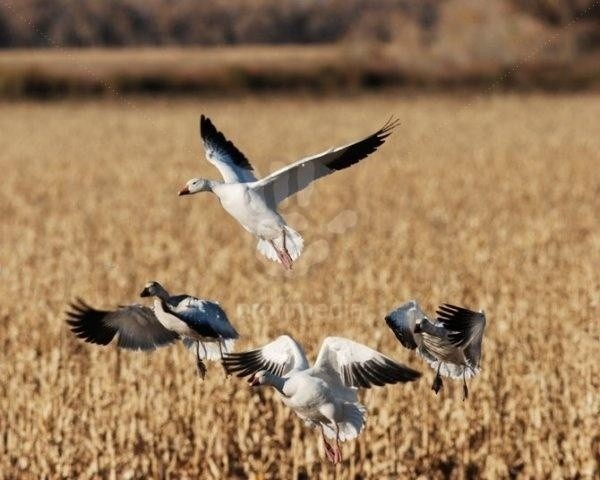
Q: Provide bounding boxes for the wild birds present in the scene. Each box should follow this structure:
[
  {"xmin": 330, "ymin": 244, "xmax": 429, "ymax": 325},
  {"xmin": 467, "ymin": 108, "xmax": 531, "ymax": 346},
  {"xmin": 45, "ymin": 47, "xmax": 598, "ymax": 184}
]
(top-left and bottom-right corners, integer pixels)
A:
[
  {"xmin": 60, "ymin": 278, "xmax": 243, "ymax": 383},
  {"xmin": 179, "ymin": 112, "xmax": 405, "ymax": 273},
  {"xmin": 217, "ymin": 330, "xmax": 424, "ymax": 464},
  {"xmin": 383, "ymin": 299, "xmax": 487, "ymax": 401}
]
